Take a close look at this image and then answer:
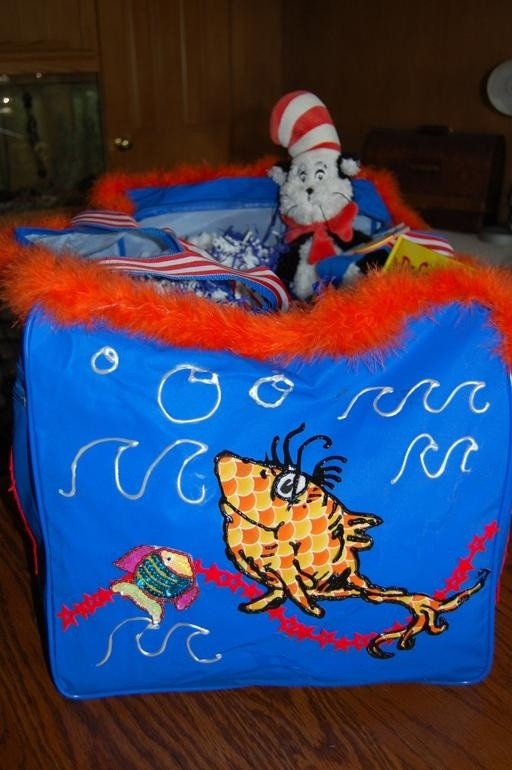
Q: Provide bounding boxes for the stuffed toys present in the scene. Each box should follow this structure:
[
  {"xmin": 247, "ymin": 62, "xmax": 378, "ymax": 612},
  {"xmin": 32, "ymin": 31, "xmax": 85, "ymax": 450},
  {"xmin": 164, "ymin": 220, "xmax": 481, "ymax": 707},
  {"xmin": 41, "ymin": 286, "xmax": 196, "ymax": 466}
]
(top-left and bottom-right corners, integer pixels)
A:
[{"xmin": 266, "ymin": 87, "xmax": 396, "ymax": 302}]
